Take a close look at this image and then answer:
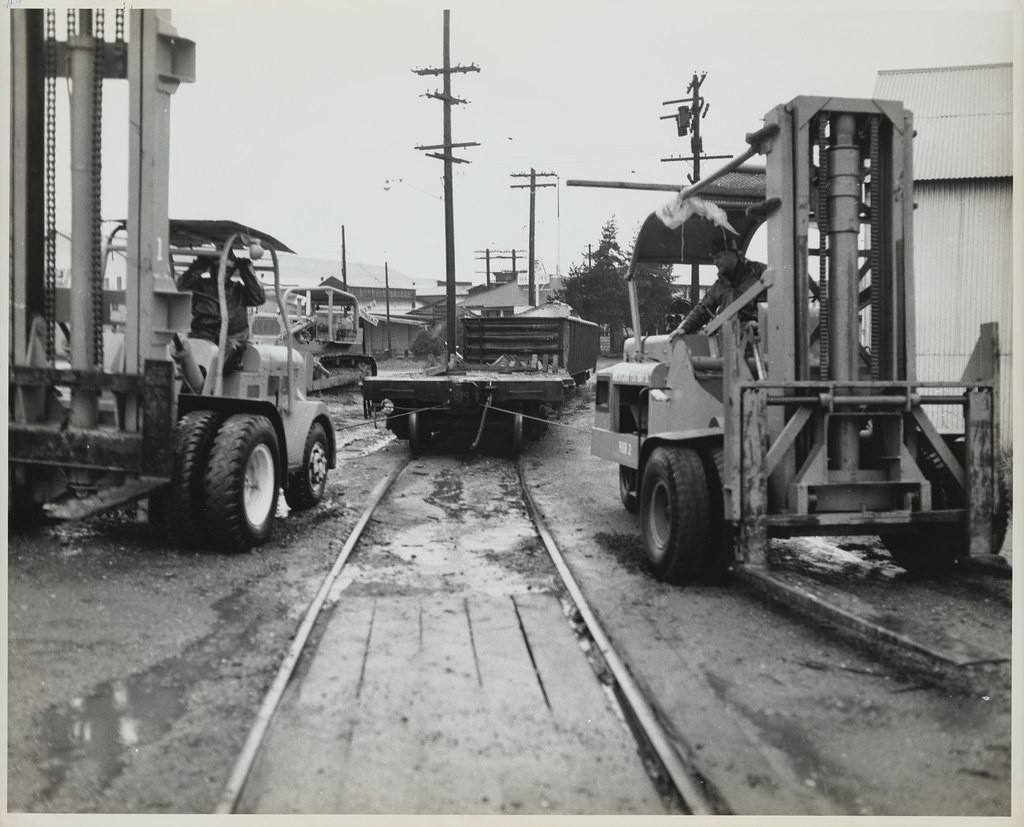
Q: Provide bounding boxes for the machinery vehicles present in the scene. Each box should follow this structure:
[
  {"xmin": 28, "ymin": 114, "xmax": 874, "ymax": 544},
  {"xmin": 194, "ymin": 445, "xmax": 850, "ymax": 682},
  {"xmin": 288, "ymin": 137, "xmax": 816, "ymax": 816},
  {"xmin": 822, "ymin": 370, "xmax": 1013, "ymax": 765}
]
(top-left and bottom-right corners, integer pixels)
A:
[
  {"xmin": 10, "ymin": 9, "xmax": 335, "ymax": 553},
  {"xmin": 250, "ymin": 286, "xmax": 378, "ymax": 398},
  {"xmin": 591, "ymin": 93, "xmax": 1009, "ymax": 588}
]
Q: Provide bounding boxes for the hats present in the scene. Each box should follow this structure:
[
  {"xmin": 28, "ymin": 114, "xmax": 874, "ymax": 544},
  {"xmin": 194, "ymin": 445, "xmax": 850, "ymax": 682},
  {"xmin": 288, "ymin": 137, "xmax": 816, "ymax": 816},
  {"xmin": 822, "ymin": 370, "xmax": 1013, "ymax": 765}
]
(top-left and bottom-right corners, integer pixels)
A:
[
  {"xmin": 210, "ymin": 245, "xmax": 236, "ymax": 268},
  {"xmin": 707, "ymin": 239, "xmax": 738, "ymax": 254}
]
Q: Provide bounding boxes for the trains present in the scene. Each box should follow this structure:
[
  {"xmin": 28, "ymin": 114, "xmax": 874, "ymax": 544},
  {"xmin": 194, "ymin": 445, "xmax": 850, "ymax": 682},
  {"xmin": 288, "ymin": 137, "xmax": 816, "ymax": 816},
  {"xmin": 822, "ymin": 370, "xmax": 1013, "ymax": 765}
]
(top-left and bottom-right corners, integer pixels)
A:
[{"xmin": 360, "ymin": 315, "xmax": 602, "ymax": 459}]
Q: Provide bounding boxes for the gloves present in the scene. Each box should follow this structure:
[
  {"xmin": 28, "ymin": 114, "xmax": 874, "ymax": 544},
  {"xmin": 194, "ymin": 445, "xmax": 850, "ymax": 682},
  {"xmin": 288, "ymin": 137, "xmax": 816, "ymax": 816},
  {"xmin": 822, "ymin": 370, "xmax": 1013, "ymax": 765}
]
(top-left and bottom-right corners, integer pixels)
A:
[
  {"xmin": 196, "ymin": 256, "xmax": 210, "ymax": 271},
  {"xmin": 235, "ymin": 257, "xmax": 251, "ymax": 269}
]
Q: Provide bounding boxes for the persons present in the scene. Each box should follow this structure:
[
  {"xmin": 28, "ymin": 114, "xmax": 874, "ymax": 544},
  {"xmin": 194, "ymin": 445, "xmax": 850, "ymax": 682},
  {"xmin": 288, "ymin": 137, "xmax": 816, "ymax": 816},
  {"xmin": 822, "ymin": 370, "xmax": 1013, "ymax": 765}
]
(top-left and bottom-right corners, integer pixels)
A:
[
  {"xmin": 177, "ymin": 248, "xmax": 266, "ymax": 373},
  {"xmin": 668, "ymin": 239, "xmax": 767, "ymax": 358}
]
